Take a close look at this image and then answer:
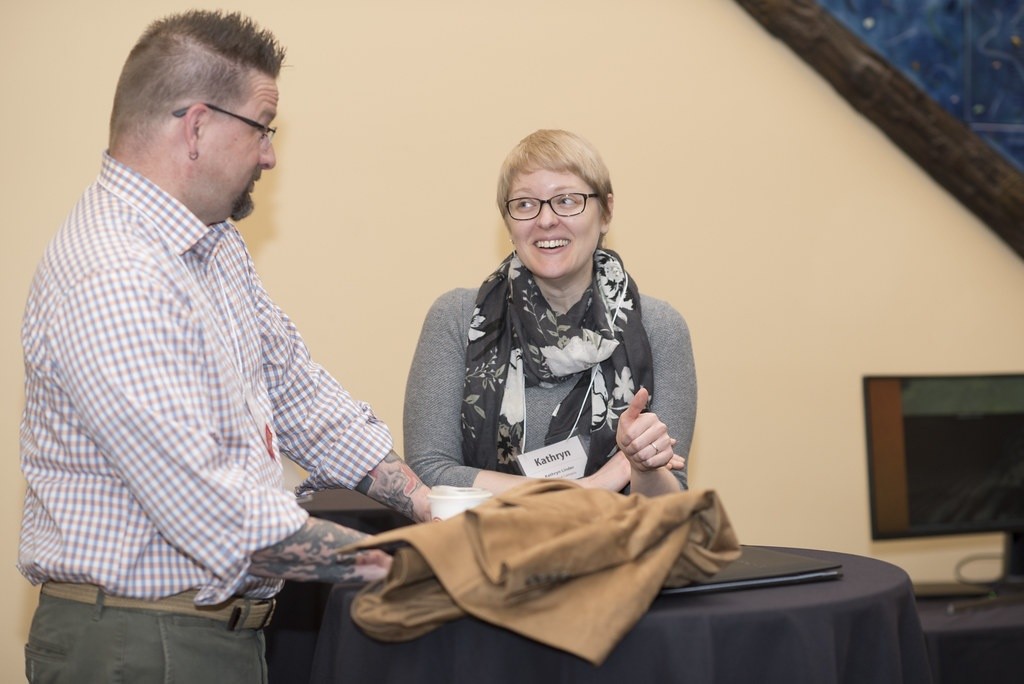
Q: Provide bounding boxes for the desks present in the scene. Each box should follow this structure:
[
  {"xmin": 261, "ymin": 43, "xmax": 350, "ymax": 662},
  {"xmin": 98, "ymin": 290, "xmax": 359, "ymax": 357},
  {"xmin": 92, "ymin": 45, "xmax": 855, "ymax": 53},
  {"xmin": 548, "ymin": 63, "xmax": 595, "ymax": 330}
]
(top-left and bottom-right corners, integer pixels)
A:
[
  {"xmin": 910, "ymin": 580, "xmax": 1024, "ymax": 684},
  {"xmin": 263, "ymin": 542, "xmax": 936, "ymax": 684}
]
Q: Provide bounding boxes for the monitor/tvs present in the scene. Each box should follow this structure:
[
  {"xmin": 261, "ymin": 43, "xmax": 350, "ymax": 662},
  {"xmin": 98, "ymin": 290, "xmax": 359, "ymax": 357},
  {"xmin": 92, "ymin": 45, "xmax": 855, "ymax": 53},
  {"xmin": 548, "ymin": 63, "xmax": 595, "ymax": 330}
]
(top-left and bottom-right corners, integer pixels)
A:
[{"xmin": 863, "ymin": 372, "xmax": 1024, "ymax": 601}]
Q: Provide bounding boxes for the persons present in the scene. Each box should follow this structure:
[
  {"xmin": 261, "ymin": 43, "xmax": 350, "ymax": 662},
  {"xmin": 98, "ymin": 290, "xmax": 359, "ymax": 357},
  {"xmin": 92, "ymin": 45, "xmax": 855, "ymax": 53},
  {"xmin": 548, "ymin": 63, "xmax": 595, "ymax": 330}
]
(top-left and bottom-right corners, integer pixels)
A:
[
  {"xmin": 18, "ymin": 10, "xmax": 436, "ymax": 684},
  {"xmin": 403, "ymin": 129, "xmax": 697, "ymax": 499}
]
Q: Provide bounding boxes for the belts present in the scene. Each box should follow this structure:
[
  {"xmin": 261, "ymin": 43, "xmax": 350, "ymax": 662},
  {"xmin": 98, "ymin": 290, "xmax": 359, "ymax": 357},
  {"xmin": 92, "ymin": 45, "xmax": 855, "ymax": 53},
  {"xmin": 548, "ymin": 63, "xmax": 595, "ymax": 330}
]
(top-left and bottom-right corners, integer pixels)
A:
[{"xmin": 40, "ymin": 580, "xmax": 279, "ymax": 628}]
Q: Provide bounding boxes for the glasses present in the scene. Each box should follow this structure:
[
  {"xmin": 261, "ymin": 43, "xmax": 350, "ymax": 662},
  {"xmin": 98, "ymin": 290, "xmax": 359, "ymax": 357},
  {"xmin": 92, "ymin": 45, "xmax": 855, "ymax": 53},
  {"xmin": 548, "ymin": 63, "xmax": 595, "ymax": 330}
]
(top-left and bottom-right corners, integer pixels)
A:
[
  {"xmin": 504, "ymin": 192, "xmax": 599, "ymax": 221},
  {"xmin": 172, "ymin": 103, "xmax": 277, "ymax": 152}
]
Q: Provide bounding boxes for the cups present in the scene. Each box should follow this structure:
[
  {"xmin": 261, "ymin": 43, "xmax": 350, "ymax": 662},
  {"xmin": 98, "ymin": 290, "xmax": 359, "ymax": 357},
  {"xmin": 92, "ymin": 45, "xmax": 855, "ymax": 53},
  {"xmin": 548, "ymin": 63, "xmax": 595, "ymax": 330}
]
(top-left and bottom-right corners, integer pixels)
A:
[{"xmin": 427, "ymin": 484, "xmax": 494, "ymax": 523}]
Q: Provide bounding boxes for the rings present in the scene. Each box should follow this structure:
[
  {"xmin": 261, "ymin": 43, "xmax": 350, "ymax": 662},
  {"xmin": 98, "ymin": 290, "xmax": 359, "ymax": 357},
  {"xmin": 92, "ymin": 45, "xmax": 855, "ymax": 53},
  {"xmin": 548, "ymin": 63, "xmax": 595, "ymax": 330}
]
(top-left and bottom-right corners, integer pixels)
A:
[{"xmin": 650, "ymin": 442, "xmax": 659, "ymax": 455}]
